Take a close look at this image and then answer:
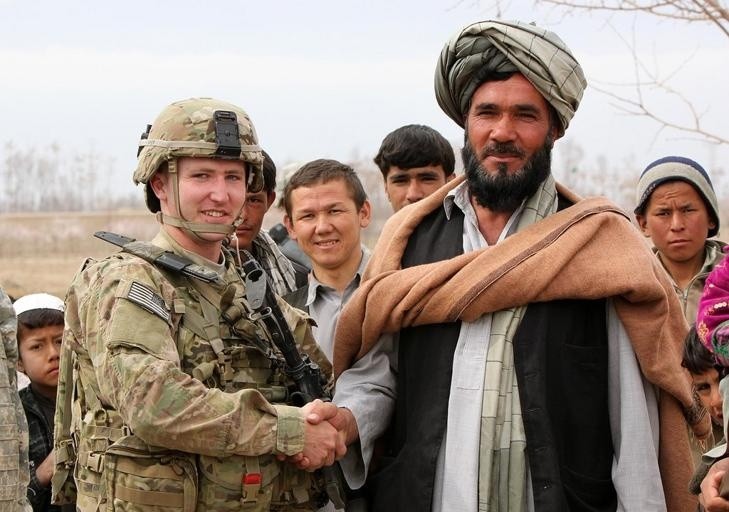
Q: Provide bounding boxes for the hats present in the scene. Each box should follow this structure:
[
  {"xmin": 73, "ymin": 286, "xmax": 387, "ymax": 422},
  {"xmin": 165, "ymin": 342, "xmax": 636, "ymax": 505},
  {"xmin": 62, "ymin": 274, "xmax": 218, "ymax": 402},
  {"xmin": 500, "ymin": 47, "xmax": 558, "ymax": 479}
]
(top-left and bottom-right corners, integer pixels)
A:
[
  {"xmin": 635, "ymin": 156, "xmax": 719, "ymax": 238},
  {"xmin": 13, "ymin": 293, "xmax": 66, "ymax": 315}
]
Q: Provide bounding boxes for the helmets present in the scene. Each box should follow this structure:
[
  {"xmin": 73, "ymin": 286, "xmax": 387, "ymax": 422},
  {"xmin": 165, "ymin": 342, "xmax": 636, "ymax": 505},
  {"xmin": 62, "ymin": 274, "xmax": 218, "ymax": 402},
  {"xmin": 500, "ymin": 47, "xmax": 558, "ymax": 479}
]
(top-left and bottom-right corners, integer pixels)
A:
[{"xmin": 134, "ymin": 97, "xmax": 265, "ymax": 193}]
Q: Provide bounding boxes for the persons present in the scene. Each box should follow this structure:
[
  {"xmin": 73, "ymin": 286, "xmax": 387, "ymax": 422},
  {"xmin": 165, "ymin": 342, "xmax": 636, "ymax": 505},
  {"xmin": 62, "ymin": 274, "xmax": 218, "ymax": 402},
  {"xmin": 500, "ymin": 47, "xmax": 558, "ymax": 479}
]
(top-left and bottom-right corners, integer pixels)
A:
[
  {"xmin": 634, "ymin": 157, "xmax": 729, "ymax": 512},
  {"xmin": 277, "ymin": 20, "xmax": 714, "ymax": 512},
  {"xmin": 51, "ymin": 98, "xmax": 347, "ymax": 512},
  {"xmin": 230, "ymin": 125, "xmax": 455, "ymax": 365},
  {"xmin": 0, "ymin": 291, "xmax": 65, "ymax": 512}
]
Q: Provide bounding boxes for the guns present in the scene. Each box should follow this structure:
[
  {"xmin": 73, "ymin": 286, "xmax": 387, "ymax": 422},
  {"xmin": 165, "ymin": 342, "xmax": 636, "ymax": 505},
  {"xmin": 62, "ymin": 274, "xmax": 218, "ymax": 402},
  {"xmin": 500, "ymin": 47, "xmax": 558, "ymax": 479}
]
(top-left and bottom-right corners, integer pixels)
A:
[{"xmin": 246, "ymin": 268, "xmax": 348, "ymax": 510}]
